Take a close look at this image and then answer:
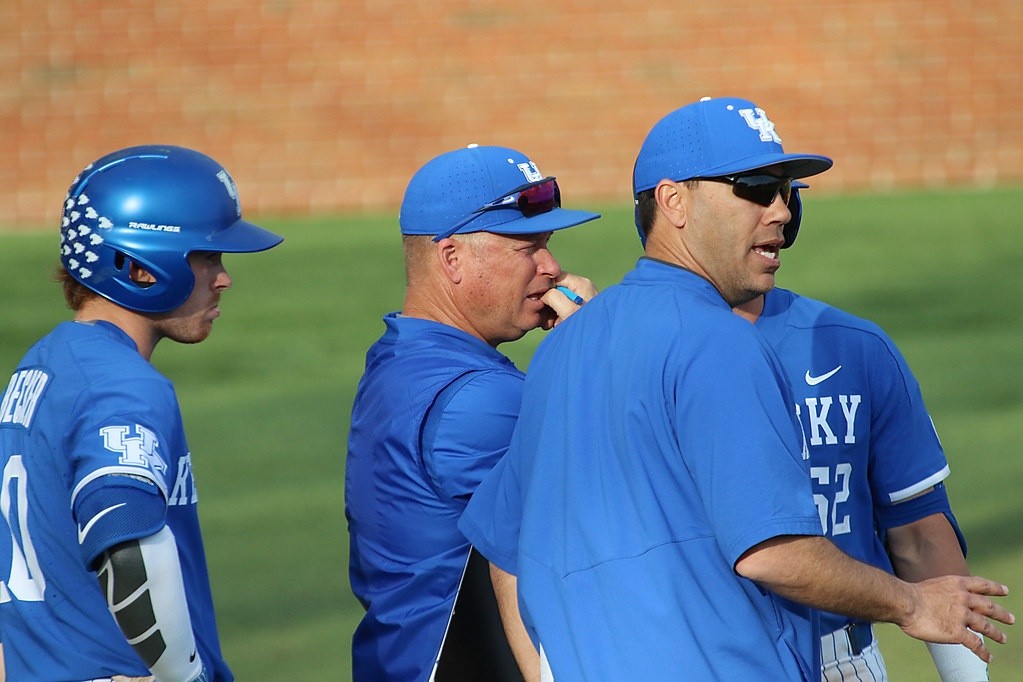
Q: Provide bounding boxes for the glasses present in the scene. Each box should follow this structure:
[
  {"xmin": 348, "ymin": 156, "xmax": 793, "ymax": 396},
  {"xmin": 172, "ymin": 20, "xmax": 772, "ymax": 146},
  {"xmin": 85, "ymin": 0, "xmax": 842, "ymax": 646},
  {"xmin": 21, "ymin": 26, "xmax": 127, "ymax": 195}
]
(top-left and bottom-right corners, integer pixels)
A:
[
  {"xmin": 431, "ymin": 176, "xmax": 561, "ymax": 243},
  {"xmin": 686, "ymin": 171, "xmax": 793, "ymax": 207}
]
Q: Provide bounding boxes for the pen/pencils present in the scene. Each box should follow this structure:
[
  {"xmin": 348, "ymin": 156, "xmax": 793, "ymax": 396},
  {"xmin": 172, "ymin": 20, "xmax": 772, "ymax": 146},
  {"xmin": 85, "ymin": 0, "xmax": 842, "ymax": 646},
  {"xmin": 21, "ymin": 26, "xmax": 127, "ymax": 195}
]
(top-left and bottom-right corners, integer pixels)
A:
[{"xmin": 557, "ymin": 286, "xmax": 583, "ymax": 305}]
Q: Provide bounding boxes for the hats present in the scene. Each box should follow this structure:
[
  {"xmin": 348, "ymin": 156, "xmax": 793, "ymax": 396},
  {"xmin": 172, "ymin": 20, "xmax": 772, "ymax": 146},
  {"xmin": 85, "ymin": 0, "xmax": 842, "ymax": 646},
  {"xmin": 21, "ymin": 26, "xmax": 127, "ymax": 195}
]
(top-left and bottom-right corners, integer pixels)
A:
[
  {"xmin": 633, "ymin": 97, "xmax": 833, "ymax": 194},
  {"xmin": 399, "ymin": 144, "xmax": 601, "ymax": 235}
]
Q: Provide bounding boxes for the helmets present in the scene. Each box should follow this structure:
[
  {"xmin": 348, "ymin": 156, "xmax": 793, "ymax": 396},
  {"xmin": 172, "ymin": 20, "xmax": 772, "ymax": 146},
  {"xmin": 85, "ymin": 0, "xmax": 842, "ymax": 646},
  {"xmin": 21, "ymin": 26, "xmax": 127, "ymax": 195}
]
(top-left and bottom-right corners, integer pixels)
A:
[
  {"xmin": 634, "ymin": 181, "xmax": 810, "ymax": 252},
  {"xmin": 59, "ymin": 145, "xmax": 285, "ymax": 312}
]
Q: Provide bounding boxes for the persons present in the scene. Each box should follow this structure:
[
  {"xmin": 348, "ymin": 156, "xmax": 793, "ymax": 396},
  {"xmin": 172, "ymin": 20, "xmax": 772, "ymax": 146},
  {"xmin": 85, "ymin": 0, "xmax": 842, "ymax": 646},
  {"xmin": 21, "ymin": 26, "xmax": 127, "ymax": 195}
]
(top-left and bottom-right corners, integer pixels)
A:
[
  {"xmin": 728, "ymin": 174, "xmax": 994, "ymax": 680},
  {"xmin": 0, "ymin": 142, "xmax": 285, "ymax": 681},
  {"xmin": 341, "ymin": 142, "xmax": 604, "ymax": 681},
  {"xmin": 456, "ymin": 95, "xmax": 1017, "ymax": 681}
]
